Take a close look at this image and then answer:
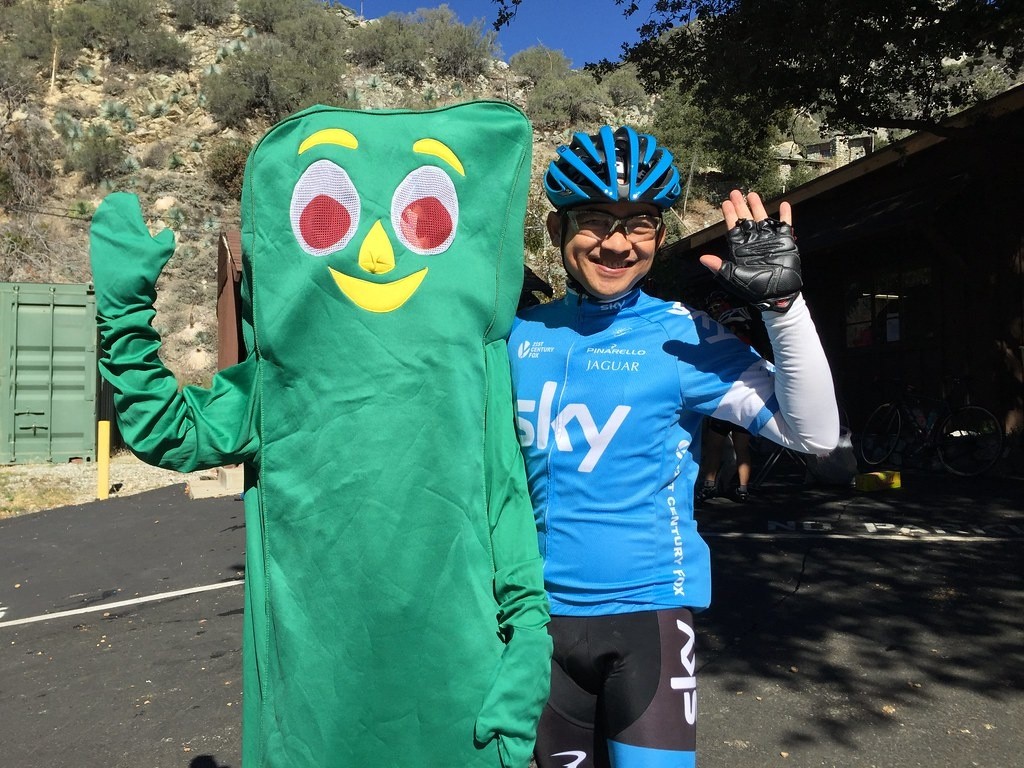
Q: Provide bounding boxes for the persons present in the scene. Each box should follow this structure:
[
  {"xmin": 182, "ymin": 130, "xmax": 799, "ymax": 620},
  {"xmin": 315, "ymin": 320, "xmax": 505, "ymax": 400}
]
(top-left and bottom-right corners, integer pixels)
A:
[
  {"xmin": 689, "ymin": 306, "xmax": 761, "ymax": 502},
  {"xmin": 88, "ymin": 97, "xmax": 553, "ymax": 768},
  {"xmin": 503, "ymin": 124, "xmax": 841, "ymax": 768}
]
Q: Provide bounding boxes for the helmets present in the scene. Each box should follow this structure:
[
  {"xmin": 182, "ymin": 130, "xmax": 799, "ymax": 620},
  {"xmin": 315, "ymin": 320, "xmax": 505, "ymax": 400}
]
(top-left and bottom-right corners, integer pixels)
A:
[{"xmin": 542, "ymin": 126, "xmax": 682, "ymax": 210}]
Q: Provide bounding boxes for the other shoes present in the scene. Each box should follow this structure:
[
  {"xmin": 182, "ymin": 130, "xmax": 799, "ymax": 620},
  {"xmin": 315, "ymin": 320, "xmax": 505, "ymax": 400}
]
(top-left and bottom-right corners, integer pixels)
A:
[
  {"xmin": 694, "ymin": 486, "xmax": 717, "ymax": 503},
  {"xmin": 734, "ymin": 486, "xmax": 747, "ymax": 501}
]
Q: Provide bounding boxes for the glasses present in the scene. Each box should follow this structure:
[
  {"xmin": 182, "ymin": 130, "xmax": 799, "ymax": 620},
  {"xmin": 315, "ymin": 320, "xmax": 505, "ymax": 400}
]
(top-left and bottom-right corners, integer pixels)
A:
[{"xmin": 560, "ymin": 205, "xmax": 663, "ymax": 243}]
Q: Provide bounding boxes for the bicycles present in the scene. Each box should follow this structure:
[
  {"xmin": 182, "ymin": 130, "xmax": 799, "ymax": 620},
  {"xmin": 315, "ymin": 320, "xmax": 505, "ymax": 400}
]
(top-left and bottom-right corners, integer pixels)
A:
[
  {"xmin": 694, "ymin": 405, "xmax": 850, "ymax": 498},
  {"xmin": 859, "ymin": 369, "xmax": 1004, "ymax": 478}
]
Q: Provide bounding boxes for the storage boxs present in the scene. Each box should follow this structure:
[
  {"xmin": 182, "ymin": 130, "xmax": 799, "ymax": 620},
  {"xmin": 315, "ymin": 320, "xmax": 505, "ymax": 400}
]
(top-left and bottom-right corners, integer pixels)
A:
[
  {"xmin": 856, "ymin": 470, "xmax": 901, "ymax": 492},
  {"xmin": 0, "ymin": 281, "xmax": 121, "ymax": 465}
]
